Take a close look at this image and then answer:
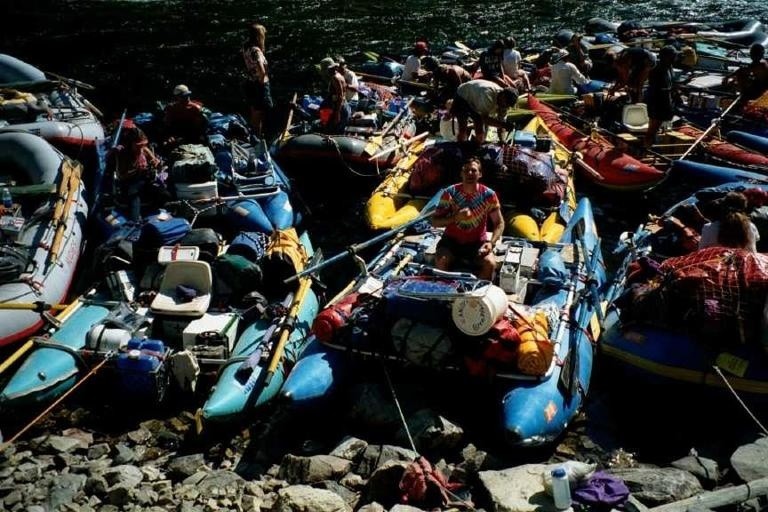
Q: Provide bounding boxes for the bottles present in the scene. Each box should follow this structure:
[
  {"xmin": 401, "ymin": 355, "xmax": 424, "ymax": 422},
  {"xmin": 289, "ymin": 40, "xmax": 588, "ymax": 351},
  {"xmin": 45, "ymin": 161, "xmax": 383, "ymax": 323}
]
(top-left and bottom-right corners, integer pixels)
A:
[
  {"xmin": 551, "ymin": 467, "xmax": 572, "ymax": 510},
  {"xmin": 2, "ymin": 188, "xmax": 12, "ymax": 209}
]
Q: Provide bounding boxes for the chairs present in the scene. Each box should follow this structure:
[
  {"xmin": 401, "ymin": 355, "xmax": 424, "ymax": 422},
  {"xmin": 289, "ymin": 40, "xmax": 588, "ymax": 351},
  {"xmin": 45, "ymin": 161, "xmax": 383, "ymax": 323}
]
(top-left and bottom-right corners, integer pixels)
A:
[
  {"xmin": 151, "ymin": 262, "xmax": 212, "ymax": 316},
  {"xmin": 622, "ymin": 101, "xmax": 673, "ymax": 169}
]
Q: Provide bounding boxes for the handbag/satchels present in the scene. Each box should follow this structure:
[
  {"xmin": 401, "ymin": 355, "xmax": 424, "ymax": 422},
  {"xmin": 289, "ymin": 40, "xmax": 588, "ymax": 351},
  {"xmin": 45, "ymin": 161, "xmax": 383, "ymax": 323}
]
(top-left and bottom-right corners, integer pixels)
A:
[{"xmin": 106, "ymin": 268, "xmax": 138, "ymax": 302}]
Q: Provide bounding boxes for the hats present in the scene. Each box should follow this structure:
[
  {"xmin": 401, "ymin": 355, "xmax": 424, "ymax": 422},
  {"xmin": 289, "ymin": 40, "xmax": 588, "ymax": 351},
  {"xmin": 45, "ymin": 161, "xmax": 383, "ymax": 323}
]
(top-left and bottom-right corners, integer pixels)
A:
[
  {"xmin": 659, "ymin": 45, "xmax": 684, "ymax": 58},
  {"xmin": 172, "ymin": 84, "xmax": 192, "ymax": 98},
  {"xmin": 414, "ymin": 42, "xmax": 429, "ymax": 54}
]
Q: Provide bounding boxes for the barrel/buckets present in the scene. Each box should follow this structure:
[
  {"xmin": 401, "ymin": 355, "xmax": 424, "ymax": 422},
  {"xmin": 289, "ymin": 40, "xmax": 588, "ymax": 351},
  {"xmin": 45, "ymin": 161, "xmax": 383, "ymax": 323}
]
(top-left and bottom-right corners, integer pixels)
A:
[{"xmin": 453, "ymin": 283, "xmax": 508, "ymax": 336}]
